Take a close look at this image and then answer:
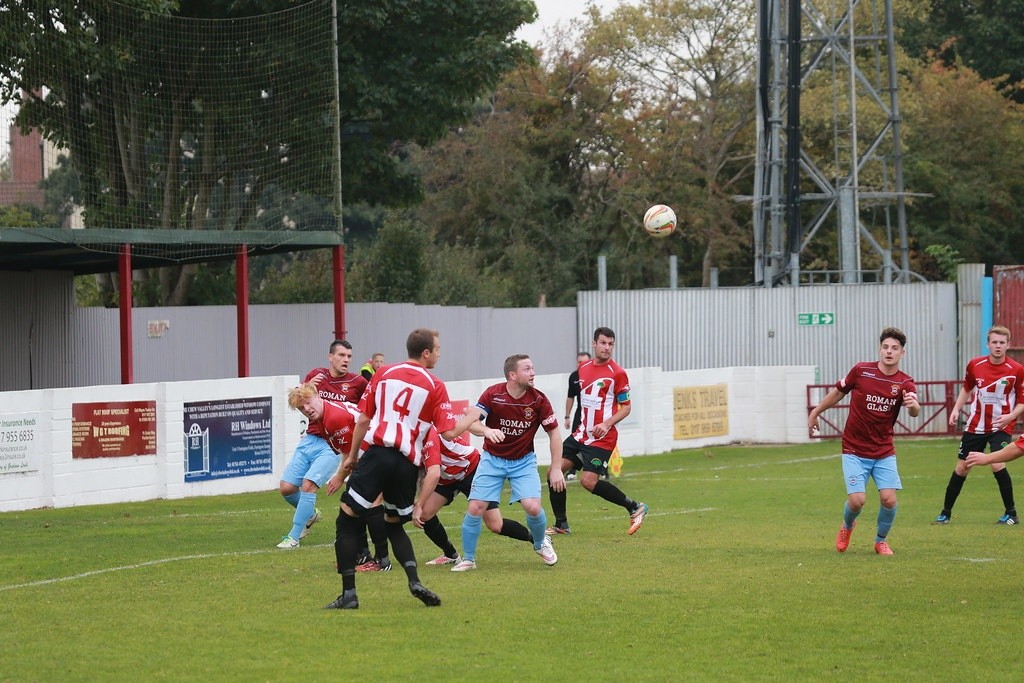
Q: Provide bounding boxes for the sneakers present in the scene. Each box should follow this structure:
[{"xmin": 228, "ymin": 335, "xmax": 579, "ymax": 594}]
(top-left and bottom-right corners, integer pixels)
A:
[
  {"xmin": 277, "ymin": 535, "xmax": 300, "ymax": 548},
  {"xmin": 547, "ymin": 525, "xmax": 570, "ymax": 536},
  {"xmin": 450, "ymin": 559, "xmax": 476, "ymax": 571},
  {"xmin": 996, "ymin": 514, "xmax": 1019, "ymax": 525},
  {"xmin": 424, "ymin": 555, "xmax": 461, "ymax": 564},
  {"xmin": 409, "ymin": 581, "xmax": 441, "ymax": 607},
  {"xmin": 336, "ymin": 555, "xmax": 373, "ymax": 572},
  {"xmin": 355, "ymin": 557, "xmax": 393, "ymax": 572},
  {"xmin": 628, "ymin": 502, "xmax": 649, "ymax": 534},
  {"xmin": 837, "ymin": 519, "xmax": 856, "ymax": 552},
  {"xmin": 325, "ymin": 588, "xmax": 359, "ymax": 610},
  {"xmin": 874, "ymin": 542, "xmax": 892, "ymax": 555},
  {"xmin": 936, "ymin": 515, "xmax": 951, "ymax": 524},
  {"xmin": 536, "ymin": 544, "xmax": 558, "ymax": 565},
  {"xmin": 546, "ymin": 535, "xmax": 551, "ymax": 545},
  {"xmin": 299, "ymin": 508, "xmax": 321, "ymax": 538}
]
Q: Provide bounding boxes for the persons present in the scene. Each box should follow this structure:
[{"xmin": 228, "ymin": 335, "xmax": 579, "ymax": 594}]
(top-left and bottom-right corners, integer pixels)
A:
[
  {"xmin": 935, "ymin": 325, "xmax": 1024, "ymax": 525},
  {"xmin": 564, "ymin": 351, "xmax": 611, "ymax": 481},
  {"xmin": 965, "ymin": 432, "xmax": 1024, "ymax": 467},
  {"xmin": 284, "ymin": 381, "xmax": 393, "ymax": 572},
  {"xmin": 806, "ymin": 327, "xmax": 920, "ymax": 555},
  {"xmin": 323, "ymin": 327, "xmax": 482, "ymax": 609},
  {"xmin": 408, "ymin": 422, "xmax": 554, "ymax": 567},
  {"xmin": 448, "ymin": 354, "xmax": 567, "ymax": 571},
  {"xmin": 360, "ymin": 353, "xmax": 384, "ymax": 382},
  {"xmin": 277, "ymin": 340, "xmax": 368, "ymax": 548},
  {"xmin": 544, "ymin": 324, "xmax": 649, "ymax": 536}
]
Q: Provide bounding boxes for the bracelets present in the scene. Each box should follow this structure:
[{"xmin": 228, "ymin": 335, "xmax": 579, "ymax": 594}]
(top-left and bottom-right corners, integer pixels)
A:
[{"xmin": 565, "ymin": 416, "xmax": 570, "ymax": 419}]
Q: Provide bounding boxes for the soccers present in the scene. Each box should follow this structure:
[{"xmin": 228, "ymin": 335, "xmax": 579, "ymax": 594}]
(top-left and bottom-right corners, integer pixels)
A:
[{"xmin": 642, "ymin": 204, "xmax": 678, "ymax": 240}]
